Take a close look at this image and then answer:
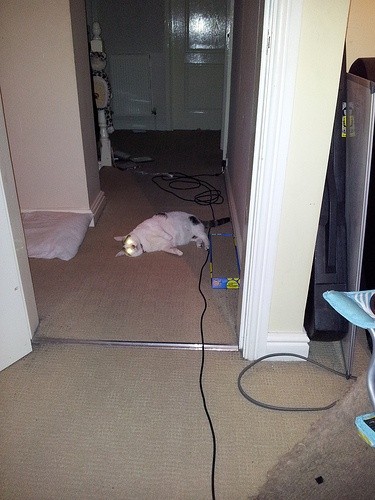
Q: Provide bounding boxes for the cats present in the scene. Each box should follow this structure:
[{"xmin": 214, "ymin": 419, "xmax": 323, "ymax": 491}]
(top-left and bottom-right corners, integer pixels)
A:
[{"xmin": 114, "ymin": 211, "xmax": 231, "ymax": 258}]
